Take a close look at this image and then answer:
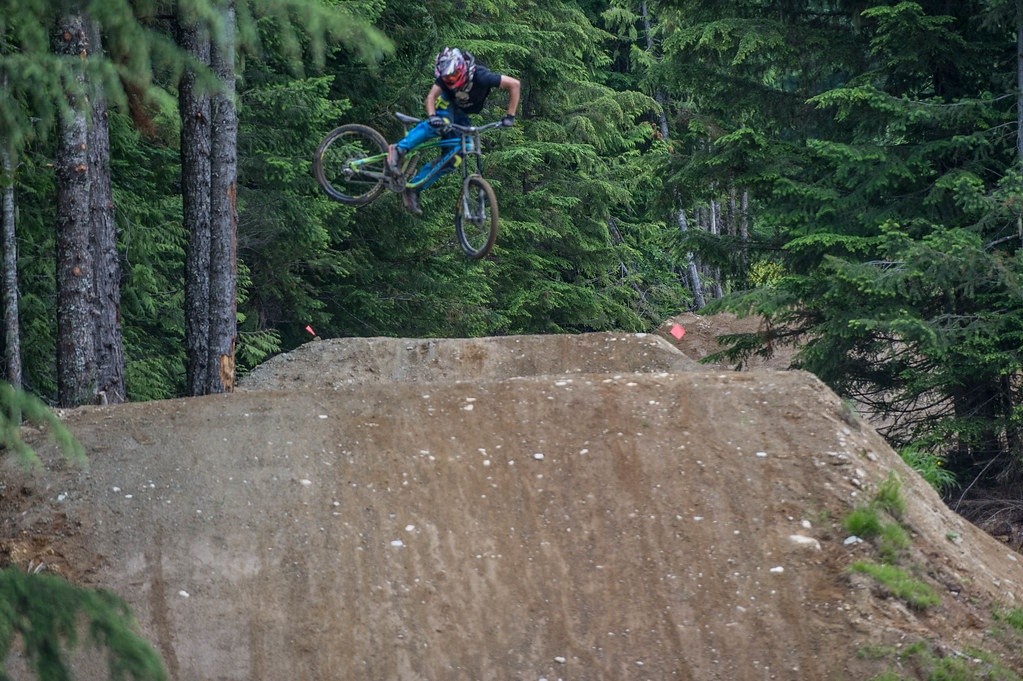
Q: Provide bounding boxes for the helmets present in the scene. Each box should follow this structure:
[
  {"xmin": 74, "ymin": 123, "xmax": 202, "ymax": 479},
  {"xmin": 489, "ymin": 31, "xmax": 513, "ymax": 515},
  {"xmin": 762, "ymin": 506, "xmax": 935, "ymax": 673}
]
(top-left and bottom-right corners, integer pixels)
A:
[{"xmin": 436, "ymin": 46, "xmax": 467, "ymax": 90}]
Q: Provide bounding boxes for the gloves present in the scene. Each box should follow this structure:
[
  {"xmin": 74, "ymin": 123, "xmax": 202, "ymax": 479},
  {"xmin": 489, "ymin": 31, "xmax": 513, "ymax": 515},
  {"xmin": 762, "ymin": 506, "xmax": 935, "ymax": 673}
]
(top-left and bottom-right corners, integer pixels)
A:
[
  {"xmin": 429, "ymin": 114, "xmax": 444, "ymax": 127},
  {"xmin": 501, "ymin": 113, "xmax": 516, "ymax": 127}
]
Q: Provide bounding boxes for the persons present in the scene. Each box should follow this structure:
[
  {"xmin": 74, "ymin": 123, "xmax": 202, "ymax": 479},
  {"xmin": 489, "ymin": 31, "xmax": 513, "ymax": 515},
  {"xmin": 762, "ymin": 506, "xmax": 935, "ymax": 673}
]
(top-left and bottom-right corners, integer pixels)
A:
[{"xmin": 387, "ymin": 46, "xmax": 519, "ymax": 216}]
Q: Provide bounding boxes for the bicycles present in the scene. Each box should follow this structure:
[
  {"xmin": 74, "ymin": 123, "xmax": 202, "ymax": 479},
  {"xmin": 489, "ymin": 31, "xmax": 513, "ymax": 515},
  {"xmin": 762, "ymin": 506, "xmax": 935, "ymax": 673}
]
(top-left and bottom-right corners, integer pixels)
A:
[{"xmin": 314, "ymin": 112, "xmax": 514, "ymax": 260}]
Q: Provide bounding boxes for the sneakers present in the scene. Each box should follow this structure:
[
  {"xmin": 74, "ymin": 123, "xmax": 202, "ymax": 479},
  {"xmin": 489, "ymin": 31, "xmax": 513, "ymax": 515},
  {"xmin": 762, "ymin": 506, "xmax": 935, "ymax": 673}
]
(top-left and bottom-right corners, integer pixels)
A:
[
  {"xmin": 387, "ymin": 144, "xmax": 403, "ymax": 177},
  {"xmin": 403, "ymin": 190, "xmax": 424, "ymax": 218}
]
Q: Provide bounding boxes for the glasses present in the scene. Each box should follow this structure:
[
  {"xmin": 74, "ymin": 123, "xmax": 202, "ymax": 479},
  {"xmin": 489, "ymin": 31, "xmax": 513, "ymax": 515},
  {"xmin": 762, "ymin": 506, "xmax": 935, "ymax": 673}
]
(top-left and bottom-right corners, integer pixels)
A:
[{"xmin": 442, "ymin": 69, "xmax": 459, "ymax": 83}]
